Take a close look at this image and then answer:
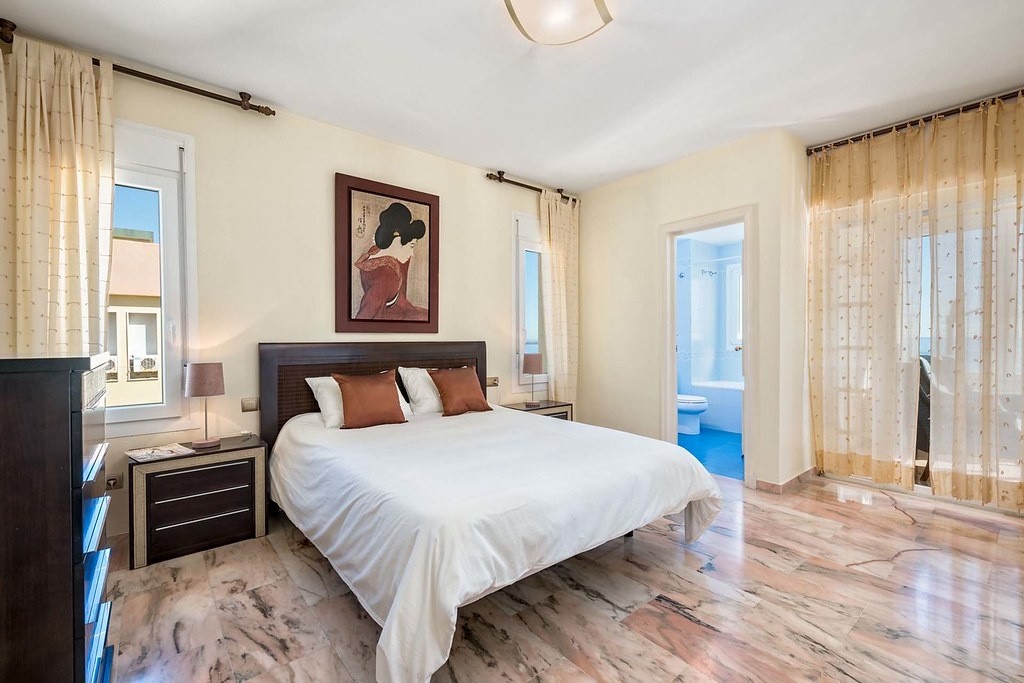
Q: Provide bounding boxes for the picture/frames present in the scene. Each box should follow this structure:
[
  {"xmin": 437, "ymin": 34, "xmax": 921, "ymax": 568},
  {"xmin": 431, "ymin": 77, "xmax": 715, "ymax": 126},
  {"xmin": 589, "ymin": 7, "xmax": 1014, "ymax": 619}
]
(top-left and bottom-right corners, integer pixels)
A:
[{"xmin": 334, "ymin": 171, "xmax": 439, "ymax": 334}]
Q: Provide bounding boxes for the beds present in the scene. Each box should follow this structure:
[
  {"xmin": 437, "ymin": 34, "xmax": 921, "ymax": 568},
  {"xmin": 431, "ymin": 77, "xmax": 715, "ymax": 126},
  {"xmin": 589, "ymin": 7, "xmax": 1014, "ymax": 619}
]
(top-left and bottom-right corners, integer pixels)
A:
[{"xmin": 258, "ymin": 340, "xmax": 722, "ymax": 682}]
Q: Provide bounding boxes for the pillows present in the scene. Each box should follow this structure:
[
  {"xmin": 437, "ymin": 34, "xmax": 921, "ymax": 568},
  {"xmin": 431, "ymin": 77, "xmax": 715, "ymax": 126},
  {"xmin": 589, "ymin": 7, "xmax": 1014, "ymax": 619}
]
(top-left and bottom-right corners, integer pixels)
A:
[
  {"xmin": 329, "ymin": 368, "xmax": 410, "ymax": 430},
  {"xmin": 427, "ymin": 366, "xmax": 494, "ymax": 417},
  {"xmin": 397, "ymin": 365, "xmax": 468, "ymax": 415},
  {"xmin": 304, "ymin": 370, "xmax": 413, "ymax": 428}
]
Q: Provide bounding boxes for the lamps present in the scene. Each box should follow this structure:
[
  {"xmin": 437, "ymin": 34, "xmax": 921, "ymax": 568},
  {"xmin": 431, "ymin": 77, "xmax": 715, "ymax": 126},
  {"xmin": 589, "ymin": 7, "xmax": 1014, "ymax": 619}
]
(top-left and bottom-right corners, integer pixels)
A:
[
  {"xmin": 184, "ymin": 362, "xmax": 225, "ymax": 449},
  {"xmin": 503, "ymin": 0, "xmax": 614, "ymax": 46},
  {"xmin": 522, "ymin": 353, "xmax": 543, "ymax": 406}
]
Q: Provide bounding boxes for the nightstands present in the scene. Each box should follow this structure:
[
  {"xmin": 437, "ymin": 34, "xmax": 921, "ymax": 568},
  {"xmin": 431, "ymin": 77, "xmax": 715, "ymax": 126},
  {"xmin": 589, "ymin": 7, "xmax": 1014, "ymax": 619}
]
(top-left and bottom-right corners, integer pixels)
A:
[
  {"xmin": 129, "ymin": 433, "xmax": 270, "ymax": 571},
  {"xmin": 500, "ymin": 400, "xmax": 573, "ymax": 421}
]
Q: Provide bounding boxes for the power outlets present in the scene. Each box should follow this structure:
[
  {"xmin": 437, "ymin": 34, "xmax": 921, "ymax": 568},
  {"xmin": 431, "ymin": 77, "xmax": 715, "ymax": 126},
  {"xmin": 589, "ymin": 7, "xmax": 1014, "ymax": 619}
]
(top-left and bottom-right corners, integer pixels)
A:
[
  {"xmin": 105, "ymin": 472, "xmax": 124, "ymax": 489},
  {"xmin": 486, "ymin": 376, "xmax": 499, "ymax": 386}
]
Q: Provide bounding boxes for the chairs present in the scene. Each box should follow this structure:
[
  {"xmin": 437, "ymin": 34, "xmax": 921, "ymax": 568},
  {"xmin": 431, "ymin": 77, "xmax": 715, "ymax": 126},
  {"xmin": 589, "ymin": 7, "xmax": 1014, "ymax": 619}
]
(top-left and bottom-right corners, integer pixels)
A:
[{"xmin": 906, "ymin": 355, "xmax": 1024, "ymax": 482}]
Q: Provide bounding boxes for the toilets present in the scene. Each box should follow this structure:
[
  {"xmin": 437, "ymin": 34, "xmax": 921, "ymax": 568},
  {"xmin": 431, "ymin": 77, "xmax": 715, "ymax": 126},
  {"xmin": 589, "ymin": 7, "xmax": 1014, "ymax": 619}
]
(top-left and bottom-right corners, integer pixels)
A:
[{"xmin": 677, "ymin": 395, "xmax": 708, "ymax": 435}]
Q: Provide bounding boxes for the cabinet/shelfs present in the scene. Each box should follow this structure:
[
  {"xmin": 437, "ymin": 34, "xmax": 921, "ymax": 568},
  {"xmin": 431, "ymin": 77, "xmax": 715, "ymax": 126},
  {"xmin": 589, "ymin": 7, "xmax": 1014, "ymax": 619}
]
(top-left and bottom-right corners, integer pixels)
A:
[{"xmin": 0, "ymin": 351, "xmax": 116, "ymax": 683}]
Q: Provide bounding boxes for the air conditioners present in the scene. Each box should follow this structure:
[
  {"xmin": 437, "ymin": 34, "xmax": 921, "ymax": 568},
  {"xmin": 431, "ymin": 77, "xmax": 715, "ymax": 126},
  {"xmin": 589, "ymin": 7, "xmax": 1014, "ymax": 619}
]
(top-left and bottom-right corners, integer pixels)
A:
[
  {"xmin": 105, "ymin": 356, "xmax": 118, "ymax": 374},
  {"xmin": 133, "ymin": 354, "xmax": 159, "ymax": 374}
]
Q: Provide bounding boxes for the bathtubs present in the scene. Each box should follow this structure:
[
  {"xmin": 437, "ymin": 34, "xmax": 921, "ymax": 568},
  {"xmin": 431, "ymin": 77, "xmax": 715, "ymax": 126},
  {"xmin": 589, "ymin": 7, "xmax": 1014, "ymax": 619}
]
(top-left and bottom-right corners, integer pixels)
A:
[{"xmin": 692, "ymin": 380, "xmax": 744, "ymax": 434}]
